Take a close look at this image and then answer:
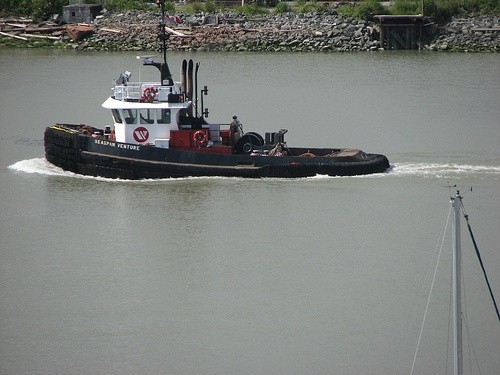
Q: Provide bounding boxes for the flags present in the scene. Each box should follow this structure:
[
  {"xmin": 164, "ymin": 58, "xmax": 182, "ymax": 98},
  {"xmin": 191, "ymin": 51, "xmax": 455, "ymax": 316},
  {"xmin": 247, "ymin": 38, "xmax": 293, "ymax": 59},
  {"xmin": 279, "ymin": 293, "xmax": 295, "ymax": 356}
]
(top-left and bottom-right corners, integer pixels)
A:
[{"xmin": 166, "ymin": 15, "xmax": 181, "ymax": 23}]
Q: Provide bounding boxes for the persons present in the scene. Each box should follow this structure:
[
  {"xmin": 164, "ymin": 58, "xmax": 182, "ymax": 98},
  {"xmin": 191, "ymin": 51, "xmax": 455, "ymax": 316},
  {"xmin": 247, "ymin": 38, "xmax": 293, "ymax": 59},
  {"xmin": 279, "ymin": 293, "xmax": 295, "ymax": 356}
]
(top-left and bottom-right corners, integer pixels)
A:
[{"xmin": 230, "ymin": 116, "xmax": 241, "ymax": 149}]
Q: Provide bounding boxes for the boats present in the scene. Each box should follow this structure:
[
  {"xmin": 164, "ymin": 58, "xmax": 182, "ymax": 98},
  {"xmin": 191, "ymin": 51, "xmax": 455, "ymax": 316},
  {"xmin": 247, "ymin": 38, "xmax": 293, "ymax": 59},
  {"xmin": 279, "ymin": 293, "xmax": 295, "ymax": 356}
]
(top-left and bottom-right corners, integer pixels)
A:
[{"xmin": 44, "ymin": 0, "xmax": 390, "ymax": 179}]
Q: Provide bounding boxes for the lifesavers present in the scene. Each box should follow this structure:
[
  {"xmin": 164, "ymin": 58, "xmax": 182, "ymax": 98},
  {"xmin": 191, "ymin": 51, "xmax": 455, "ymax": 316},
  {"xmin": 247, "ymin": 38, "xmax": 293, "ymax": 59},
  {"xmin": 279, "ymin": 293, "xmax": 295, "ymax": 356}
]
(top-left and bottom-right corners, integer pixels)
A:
[
  {"xmin": 143, "ymin": 87, "xmax": 156, "ymax": 100},
  {"xmin": 193, "ymin": 130, "xmax": 207, "ymax": 144}
]
